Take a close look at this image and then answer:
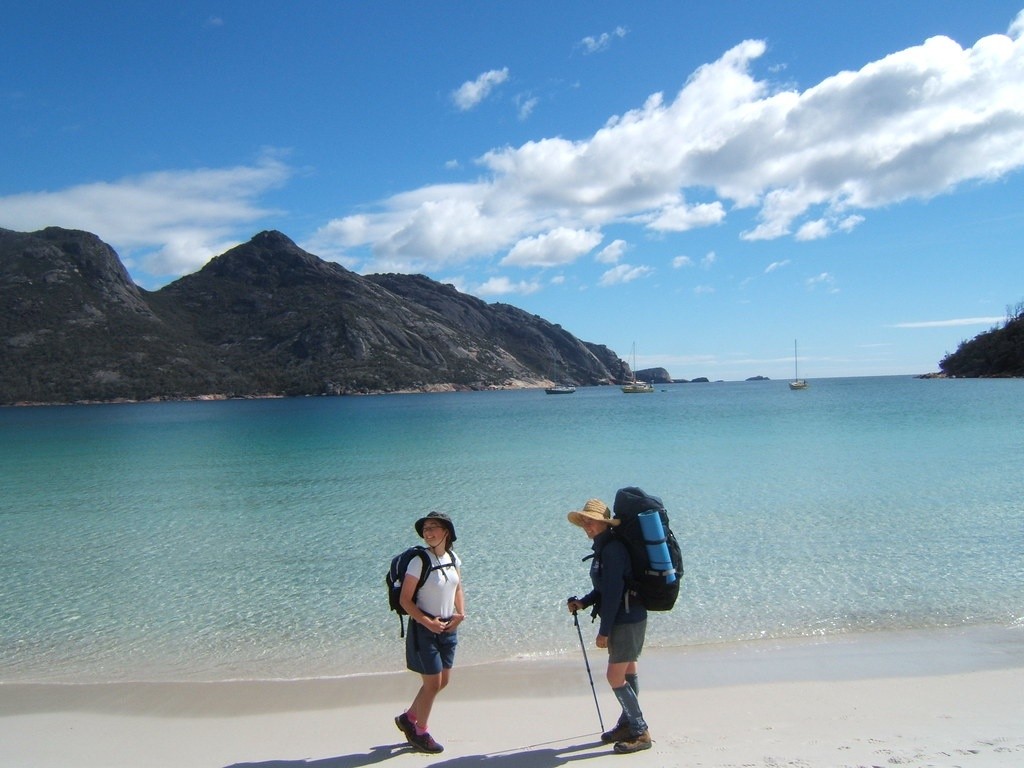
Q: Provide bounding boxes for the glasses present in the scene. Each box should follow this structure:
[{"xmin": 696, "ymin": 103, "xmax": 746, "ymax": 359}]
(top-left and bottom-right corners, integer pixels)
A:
[{"xmin": 422, "ymin": 525, "xmax": 444, "ymax": 532}]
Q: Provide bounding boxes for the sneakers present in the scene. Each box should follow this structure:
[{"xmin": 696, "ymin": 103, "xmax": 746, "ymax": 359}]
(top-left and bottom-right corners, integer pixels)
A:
[
  {"xmin": 613, "ymin": 730, "xmax": 652, "ymax": 753},
  {"xmin": 395, "ymin": 711, "xmax": 417, "ymax": 745},
  {"xmin": 405, "ymin": 727, "xmax": 444, "ymax": 753},
  {"xmin": 602, "ymin": 721, "xmax": 632, "ymax": 740}
]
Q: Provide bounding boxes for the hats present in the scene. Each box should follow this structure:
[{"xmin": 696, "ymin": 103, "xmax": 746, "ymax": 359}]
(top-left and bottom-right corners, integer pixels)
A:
[
  {"xmin": 568, "ymin": 499, "xmax": 621, "ymax": 527},
  {"xmin": 415, "ymin": 511, "xmax": 457, "ymax": 542}
]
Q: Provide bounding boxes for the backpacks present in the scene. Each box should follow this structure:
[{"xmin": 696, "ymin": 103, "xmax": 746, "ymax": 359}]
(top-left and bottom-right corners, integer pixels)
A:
[
  {"xmin": 594, "ymin": 486, "xmax": 684, "ymax": 611},
  {"xmin": 386, "ymin": 545, "xmax": 459, "ymax": 637}
]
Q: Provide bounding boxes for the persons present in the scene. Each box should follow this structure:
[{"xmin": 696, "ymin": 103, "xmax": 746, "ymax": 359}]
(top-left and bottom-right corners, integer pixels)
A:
[
  {"xmin": 394, "ymin": 511, "xmax": 466, "ymax": 754},
  {"xmin": 567, "ymin": 498, "xmax": 652, "ymax": 754}
]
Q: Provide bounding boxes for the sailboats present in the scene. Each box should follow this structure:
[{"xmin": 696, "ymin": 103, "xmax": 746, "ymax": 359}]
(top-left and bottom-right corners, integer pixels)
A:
[
  {"xmin": 544, "ymin": 348, "xmax": 576, "ymax": 394},
  {"xmin": 620, "ymin": 341, "xmax": 655, "ymax": 392},
  {"xmin": 789, "ymin": 339, "xmax": 809, "ymax": 390}
]
